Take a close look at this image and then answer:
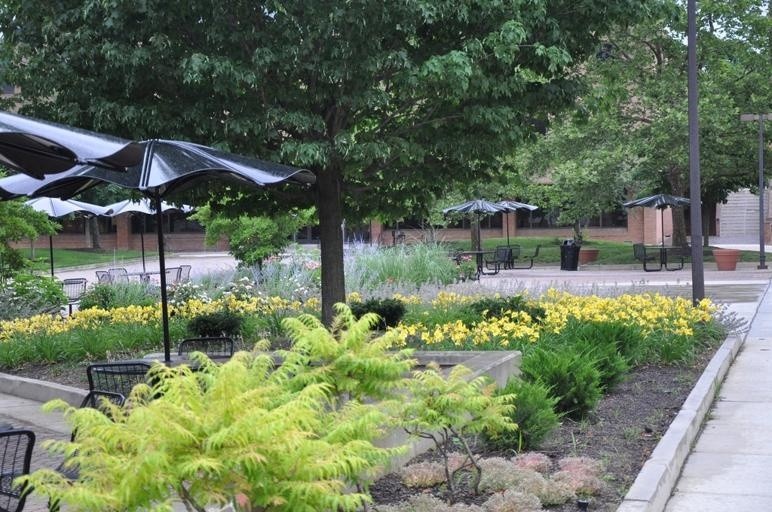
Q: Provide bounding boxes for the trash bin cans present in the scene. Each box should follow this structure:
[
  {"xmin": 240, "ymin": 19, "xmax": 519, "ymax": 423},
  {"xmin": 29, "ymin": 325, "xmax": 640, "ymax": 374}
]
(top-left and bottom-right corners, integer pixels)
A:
[{"xmin": 560, "ymin": 239, "xmax": 581, "ymax": 271}]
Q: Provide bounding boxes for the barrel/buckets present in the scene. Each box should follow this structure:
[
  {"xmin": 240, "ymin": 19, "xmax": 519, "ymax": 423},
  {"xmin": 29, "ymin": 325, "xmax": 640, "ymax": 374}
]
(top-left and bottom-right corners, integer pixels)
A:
[{"xmin": 560, "ymin": 244, "xmax": 580, "ymax": 271}]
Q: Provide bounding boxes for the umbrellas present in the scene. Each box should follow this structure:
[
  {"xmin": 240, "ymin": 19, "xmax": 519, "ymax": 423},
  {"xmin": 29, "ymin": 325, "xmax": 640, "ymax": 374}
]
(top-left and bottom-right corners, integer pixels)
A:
[
  {"xmin": 622, "ymin": 192, "xmax": 702, "ymax": 272},
  {"xmin": 443, "ymin": 200, "xmax": 510, "ymax": 275},
  {"xmin": 493, "ymin": 200, "xmax": 538, "ymax": 271},
  {"xmin": 0, "ymin": 109, "xmax": 144, "ymax": 180},
  {"xmin": 0, "ymin": 138, "xmax": 317, "ymax": 361}
]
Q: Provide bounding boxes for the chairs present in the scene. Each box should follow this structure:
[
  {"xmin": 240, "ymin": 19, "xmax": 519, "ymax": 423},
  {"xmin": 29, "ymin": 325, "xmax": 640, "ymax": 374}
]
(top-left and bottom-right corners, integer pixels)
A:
[
  {"xmin": 2, "ymin": 429, "xmax": 36, "ymax": 512},
  {"xmin": 449, "ymin": 243, "xmax": 541, "ymax": 278},
  {"xmin": 633, "ymin": 242, "xmax": 691, "ymax": 276},
  {"xmin": 40, "ymin": 337, "xmax": 236, "ymax": 511},
  {"xmin": 60, "ymin": 263, "xmax": 191, "ymax": 319}
]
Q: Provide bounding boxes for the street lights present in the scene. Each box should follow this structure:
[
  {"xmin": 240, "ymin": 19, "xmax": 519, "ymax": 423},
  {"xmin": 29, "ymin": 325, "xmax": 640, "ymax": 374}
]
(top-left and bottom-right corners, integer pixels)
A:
[{"xmin": 739, "ymin": 112, "xmax": 772, "ymax": 269}]
